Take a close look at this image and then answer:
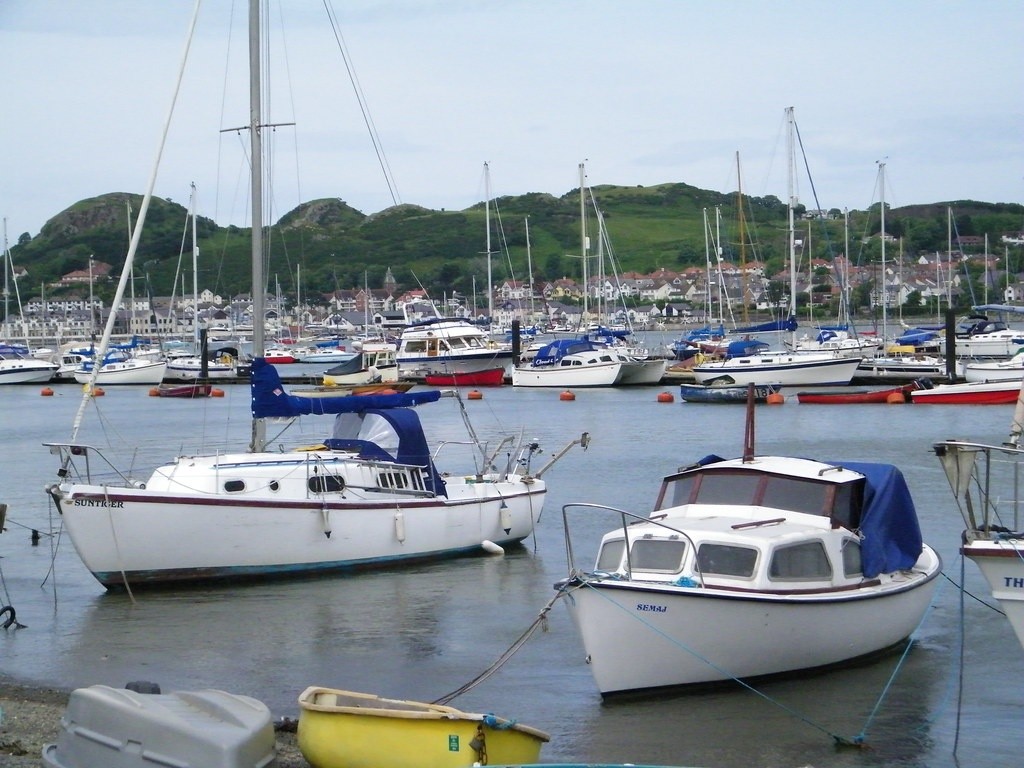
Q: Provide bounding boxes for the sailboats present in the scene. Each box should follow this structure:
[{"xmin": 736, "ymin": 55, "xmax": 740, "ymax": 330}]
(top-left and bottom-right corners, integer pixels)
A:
[{"xmin": 36, "ymin": 0, "xmax": 555, "ymax": 595}]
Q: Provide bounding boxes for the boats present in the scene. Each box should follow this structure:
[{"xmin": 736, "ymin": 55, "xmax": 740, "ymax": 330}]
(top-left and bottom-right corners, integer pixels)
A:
[
  {"xmin": 549, "ymin": 376, "xmax": 945, "ymax": 704},
  {"xmin": 295, "ymin": 687, "xmax": 550, "ymax": 768},
  {"xmin": 930, "ymin": 440, "xmax": 1024, "ymax": 651},
  {"xmin": 0, "ymin": 97, "xmax": 1024, "ymax": 409},
  {"xmin": 38, "ymin": 680, "xmax": 278, "ymax": 768}
]
